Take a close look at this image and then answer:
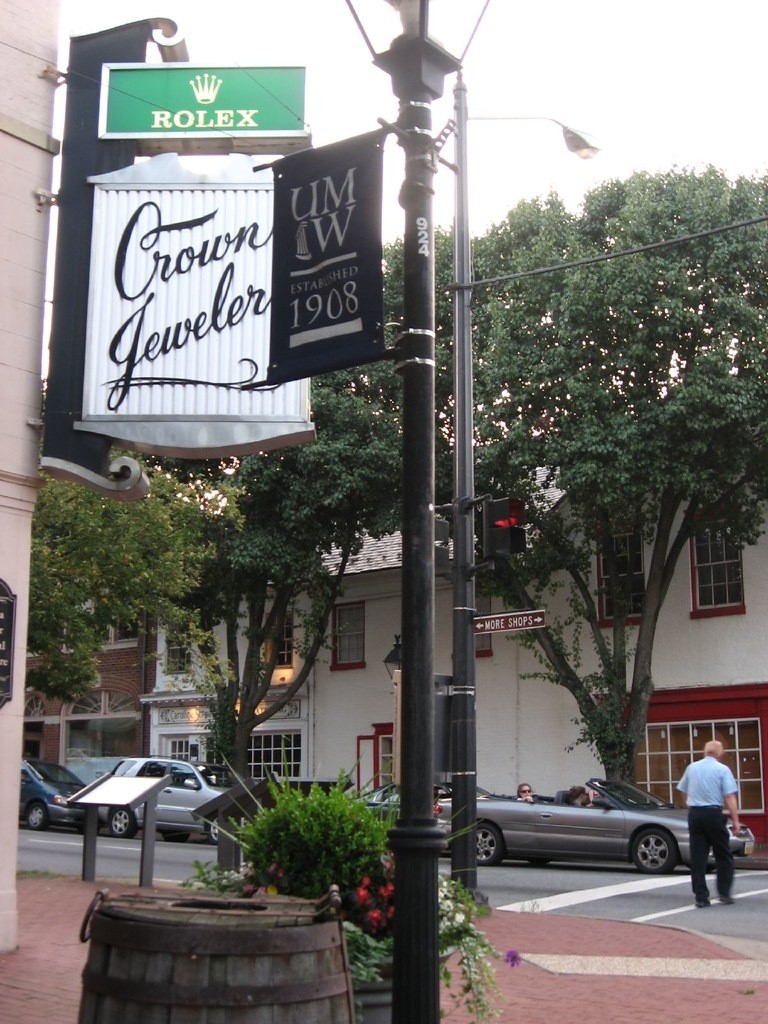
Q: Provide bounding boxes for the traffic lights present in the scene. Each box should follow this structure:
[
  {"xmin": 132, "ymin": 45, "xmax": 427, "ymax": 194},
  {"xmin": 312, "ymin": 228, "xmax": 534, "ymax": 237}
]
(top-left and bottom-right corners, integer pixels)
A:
[{"xmin": 481, "ymin": 494, "xmax": 528, "ymax": 561}]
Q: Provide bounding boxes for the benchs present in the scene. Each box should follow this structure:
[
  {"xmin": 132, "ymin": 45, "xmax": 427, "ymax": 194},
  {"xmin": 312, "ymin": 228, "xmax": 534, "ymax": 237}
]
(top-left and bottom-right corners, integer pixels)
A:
[{"xmin": 553, "ymin": 790, "xmax": 590, "ymax": 806}]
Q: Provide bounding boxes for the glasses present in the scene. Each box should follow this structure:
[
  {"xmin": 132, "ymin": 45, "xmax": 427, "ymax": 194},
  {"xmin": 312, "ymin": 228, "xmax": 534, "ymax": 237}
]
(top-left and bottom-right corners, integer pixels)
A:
[{"xmin": 519, "ymin": 789, "xmax": 531, "ymax": 793}]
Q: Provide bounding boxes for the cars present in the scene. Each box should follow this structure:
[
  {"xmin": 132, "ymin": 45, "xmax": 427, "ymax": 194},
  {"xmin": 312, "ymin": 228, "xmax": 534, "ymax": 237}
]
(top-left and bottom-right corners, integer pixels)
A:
[
  {"xmin": 18, "ymin": 758, "xmax": 93, "ymax": 834},
  {"xmin": 347, "ymin": 777, "xmax": 493, "ymax": 831},
  {"xmin": 99, "ymin": 757, "xmax": 238, "ymax": 846}
]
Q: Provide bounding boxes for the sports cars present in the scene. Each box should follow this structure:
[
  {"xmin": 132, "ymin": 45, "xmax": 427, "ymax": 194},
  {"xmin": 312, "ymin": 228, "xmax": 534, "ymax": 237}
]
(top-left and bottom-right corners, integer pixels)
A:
[{"xmin": 429, "ymin": 777, "xmax": 756, "ymax": 875}]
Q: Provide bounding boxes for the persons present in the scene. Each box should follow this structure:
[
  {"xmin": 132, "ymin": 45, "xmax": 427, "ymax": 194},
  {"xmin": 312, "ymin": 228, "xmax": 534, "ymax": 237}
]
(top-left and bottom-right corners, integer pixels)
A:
[
  {"xmin": 676, "ymin": 741, "xmax": 740, "ymax": 907},
  {"xmin": 566, "ymin": 786, "xmax": 604, "ymax": 810},
  {"xmin": 516, "ymin": 783, "xmax": 540, "ymax": 804}
]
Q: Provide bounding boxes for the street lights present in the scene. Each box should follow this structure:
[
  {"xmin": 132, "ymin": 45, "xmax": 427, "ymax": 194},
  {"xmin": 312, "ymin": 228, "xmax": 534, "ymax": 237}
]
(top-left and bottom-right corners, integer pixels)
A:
[{"xmin": 447, "ymin": 104, "xmax": 597, "ymax": 914}]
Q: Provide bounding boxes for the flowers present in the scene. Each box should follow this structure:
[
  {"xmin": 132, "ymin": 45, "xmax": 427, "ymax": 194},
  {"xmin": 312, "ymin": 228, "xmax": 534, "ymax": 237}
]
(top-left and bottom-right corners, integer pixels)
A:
[{"xmin": 179, "ymin": 733, "xmax": 522, "ymax": 1024}]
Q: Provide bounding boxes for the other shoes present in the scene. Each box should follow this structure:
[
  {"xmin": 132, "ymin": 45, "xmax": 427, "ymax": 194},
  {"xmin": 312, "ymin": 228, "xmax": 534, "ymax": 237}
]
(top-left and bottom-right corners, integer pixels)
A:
[
  {"xmin": 695, "ymin": 899, "xmax": 710, "ymax": 907},
  {"xmin": 719, "ymin": 896, "xmax": 734, "ymax": 903}
]
